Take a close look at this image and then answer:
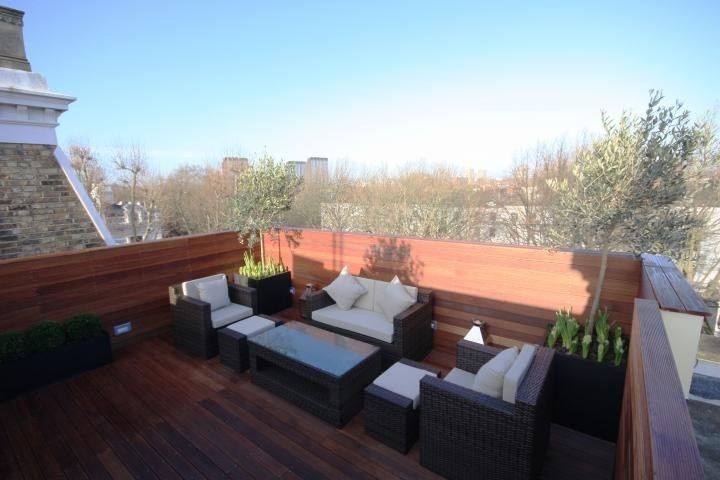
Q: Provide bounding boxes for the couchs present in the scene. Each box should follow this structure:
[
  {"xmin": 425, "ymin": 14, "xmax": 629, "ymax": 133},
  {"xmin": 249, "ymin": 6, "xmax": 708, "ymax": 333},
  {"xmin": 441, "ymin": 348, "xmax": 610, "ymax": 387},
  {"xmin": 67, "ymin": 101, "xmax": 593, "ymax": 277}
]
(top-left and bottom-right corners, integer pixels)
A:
[{"xmin": 307, "ymin": 267, "xmax": 434, "ymax": 363}]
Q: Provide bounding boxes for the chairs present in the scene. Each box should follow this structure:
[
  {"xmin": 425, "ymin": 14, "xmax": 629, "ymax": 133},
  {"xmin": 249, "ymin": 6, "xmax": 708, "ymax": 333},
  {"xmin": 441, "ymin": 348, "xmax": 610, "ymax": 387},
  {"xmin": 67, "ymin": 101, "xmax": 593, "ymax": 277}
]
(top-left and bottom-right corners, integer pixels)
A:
[
  {"xmin": 176, "ymin": 273, "xmax": 260, "ymax": 361},
  {"xmin": 418, "ymin": 338, "xmax": 555, "ymax": 479}
]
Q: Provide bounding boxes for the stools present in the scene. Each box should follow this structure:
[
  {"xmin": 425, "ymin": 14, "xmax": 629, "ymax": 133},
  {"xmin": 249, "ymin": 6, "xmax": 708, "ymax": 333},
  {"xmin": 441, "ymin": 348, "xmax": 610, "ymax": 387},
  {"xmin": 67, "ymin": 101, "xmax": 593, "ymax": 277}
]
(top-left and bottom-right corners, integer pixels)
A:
[
  {"xmin": 365, "ymin": 357, "xmax": 440, "ymax": 455},
  {"xmin": 218, "ymin": 312, "xmax": 282, "ymax": 375}
]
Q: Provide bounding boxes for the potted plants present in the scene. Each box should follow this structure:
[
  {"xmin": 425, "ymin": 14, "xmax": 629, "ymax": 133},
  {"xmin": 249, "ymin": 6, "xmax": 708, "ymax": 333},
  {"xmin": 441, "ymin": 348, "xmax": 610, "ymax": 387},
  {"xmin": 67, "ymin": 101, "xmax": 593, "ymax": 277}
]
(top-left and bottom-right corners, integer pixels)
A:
[
  {"xmin": 537, "ymin": 86, "xmax": 720, "ymax": 445},
  {"xmin": 234, "ymin": 161, "xmax": 305, "ymax": 314},
  {"xmin": 0, "ymin": 313, "xmax": 113, "ymax": 401}
]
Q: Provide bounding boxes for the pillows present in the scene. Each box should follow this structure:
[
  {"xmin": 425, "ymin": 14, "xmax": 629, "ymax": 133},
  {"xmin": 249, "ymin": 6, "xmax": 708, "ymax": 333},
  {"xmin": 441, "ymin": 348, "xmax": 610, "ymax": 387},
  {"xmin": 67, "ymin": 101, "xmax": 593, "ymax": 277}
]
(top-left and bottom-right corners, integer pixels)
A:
[
  {"xmin": 322, "ymin": 266, "xmax": 367, "ymax": 312},
  {"xmin": 196, "ymin": 278, "xmax": 231, "ymax": 310},
  {"xmin": 374, "ymin": 275, "xmax": 416, "ymax": 326},
  {"xmin": 469, "ymin": 343, "xmax": 521, "ymax": 404}
]
